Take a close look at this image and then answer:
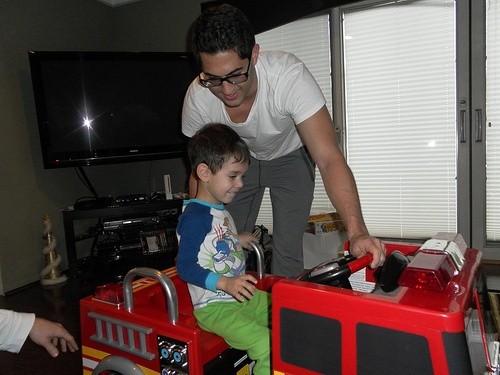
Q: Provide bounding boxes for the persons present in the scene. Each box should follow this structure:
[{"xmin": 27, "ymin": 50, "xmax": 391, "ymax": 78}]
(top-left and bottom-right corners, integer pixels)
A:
[
  {"xmin": 0, "ymin": 309, "xmax": 80, "ymax": 359},
  {"xmin": 174, "ymin": 123, "xmax": 276, "ymax": 373},
  {"xmin": 177, "ymin": 4, "xmax": 390, "ymax": 269}
]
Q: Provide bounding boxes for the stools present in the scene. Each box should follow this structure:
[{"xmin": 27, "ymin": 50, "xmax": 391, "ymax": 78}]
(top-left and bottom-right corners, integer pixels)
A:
[{"xmin": 131, "ymin": 267, "xmax": 290, "ymax": 370}]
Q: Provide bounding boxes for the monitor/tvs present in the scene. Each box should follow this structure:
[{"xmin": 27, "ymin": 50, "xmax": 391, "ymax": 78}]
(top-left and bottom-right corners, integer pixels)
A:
[{"xmin": 27, "ymin": 49, "xmax": 204, "ymax": 171}]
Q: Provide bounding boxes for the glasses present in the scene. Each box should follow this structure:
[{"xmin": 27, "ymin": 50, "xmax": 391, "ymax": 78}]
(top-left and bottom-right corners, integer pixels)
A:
[{"xmin": 197, "ymin": 57, "xmax": 251, "ymax": 89}]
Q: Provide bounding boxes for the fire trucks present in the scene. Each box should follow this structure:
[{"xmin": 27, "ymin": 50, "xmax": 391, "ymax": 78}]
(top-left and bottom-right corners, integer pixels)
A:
[{"xmin": 80, "ymin": 240, "xmax": 500, "ymax": 375}]
